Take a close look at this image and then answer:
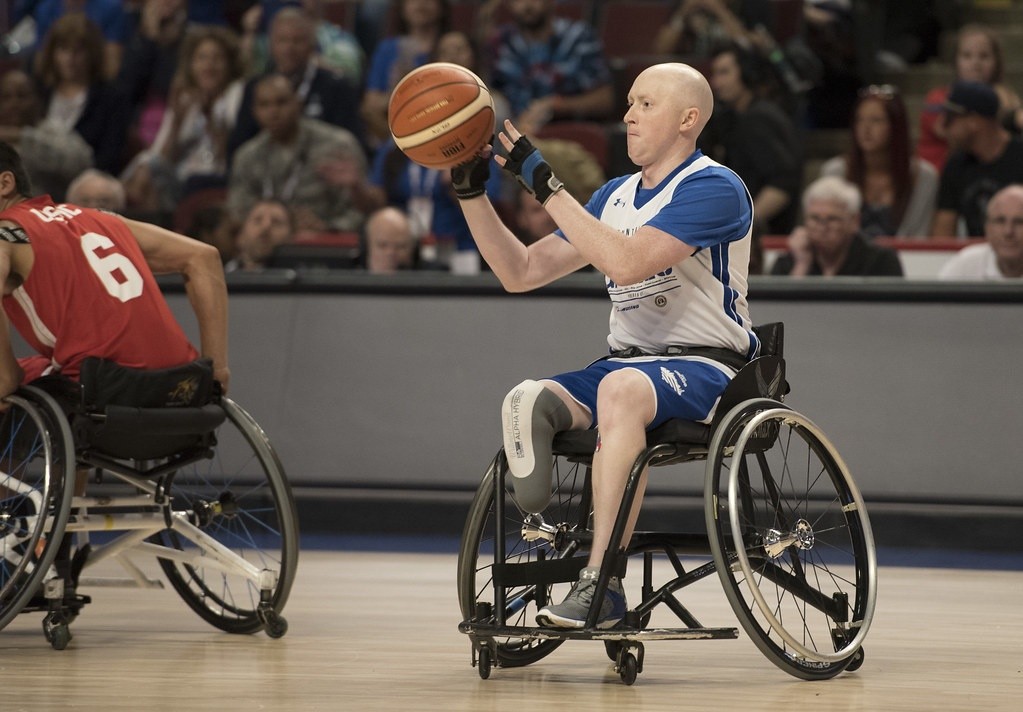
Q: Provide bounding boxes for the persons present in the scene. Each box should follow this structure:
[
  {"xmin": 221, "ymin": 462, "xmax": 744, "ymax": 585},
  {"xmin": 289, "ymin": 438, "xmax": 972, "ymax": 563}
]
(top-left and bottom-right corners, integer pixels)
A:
[
  {"xmin": 360, "ymin": 0, "xmax": 449, "ymax": 126},
  {"xmin": 931, "ymin": 83, "xmax": 1023, "ymax": 239},
  {"xmin": 917, "ymin": 28, "xmax": 1023, "ymax": 168},
  {"xmin": 478, "ymin": 142, "xmax": 606, "ymax": 275},
  {"xmin": 820, "ymin": 84, "xmax": 939, "ymax": 239},
  {"xmin": 519, "ymin": 0, "xmax": 876, "ymax": 222},
  {"xmin": 368, "ymin": 133, "xmax": 500, "ymax": 250},
  {"xmin": 0, "ymin": 141, "xmax": 230, "ymax": 501},
  {"xmin": 435, "ymin": 30, "xmax": 509, "ymax": 129},
  {"xmin": 450, "ymin": 62, "xmax": 762, "ymax": 628},
  {"xmin": 130, "ymin": 1, "xmax": 446, "ymax": 273},
  {"xmin": 0, "ymin": 0, "xmax": 133, "ymax": 200},
  {"xmin": 489, "ymin": 0, "xmax": 616, "ymax": 136},
  {"xmin": 64, "ymin": 168, "xmax": 127, "ymax": 214},
  {"xmin": 771, "ymin": 176, "xmax": 904, "ymax": 277},
  {"xmin": 939, "ymin": 185, "xmax": 1023, "ymax": 280}
]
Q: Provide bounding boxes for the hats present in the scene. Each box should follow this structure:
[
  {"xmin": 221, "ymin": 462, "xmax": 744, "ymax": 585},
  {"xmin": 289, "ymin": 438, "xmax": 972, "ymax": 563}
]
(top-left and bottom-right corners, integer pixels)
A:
[{"xmin": 927, "ymin": 79, "xmax": 1000, "ymax": 121}]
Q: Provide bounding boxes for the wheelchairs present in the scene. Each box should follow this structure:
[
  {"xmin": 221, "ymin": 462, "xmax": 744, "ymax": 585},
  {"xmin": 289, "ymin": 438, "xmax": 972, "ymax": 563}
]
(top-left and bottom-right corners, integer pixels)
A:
[
  {"xmin": 0, "ymin": 356, "xmax": 300, "ymax": 650},
  {"xmin": 457, "ymin": 321, "xmax": 877, "ymax": 686}
]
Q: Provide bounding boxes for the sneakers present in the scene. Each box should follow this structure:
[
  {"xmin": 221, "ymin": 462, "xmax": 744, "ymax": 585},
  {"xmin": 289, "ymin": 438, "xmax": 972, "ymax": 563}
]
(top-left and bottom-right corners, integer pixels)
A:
[{"xmin": 535, "ymin": 567, "xmax": 628, "ymax": 629}]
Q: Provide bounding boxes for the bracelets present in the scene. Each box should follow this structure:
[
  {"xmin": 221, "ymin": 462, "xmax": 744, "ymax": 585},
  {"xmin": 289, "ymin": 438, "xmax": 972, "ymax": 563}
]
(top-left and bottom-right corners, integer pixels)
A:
[{"xmin": 671, "ymin": 18, "xmax": 683, "ymax": 32}]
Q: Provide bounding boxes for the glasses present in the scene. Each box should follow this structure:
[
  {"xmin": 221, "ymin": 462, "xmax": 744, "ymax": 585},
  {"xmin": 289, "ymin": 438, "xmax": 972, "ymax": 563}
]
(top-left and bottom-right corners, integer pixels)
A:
[
  {"xmin": 858, "ymin": 85, "xmax": 896, "ymax": 101},
  {"xmin": 804, "ymin": 213, "xmax": 852, "ymax": 231}
]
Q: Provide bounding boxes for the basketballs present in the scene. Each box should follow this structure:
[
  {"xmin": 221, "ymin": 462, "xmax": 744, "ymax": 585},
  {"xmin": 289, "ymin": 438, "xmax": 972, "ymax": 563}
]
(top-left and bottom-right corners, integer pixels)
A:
[{"xmin": 384, "ymin": 60, "xmax": 498, "ymax": 173}]
